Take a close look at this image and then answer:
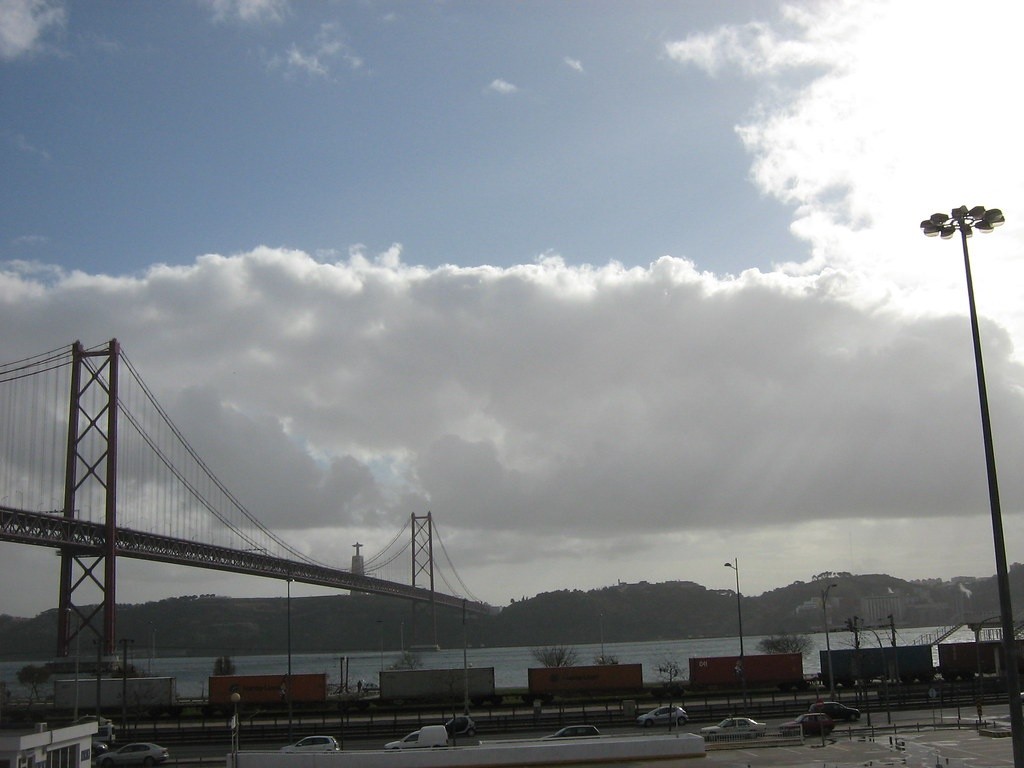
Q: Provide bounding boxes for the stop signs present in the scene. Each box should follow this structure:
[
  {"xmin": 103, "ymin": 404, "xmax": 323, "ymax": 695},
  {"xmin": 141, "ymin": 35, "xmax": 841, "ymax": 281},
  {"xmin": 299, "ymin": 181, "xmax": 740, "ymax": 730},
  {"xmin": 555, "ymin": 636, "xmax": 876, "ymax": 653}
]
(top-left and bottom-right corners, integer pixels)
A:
[{"xmin": 813, "ymin": 700, "xmax": 824, "ymax": 709}]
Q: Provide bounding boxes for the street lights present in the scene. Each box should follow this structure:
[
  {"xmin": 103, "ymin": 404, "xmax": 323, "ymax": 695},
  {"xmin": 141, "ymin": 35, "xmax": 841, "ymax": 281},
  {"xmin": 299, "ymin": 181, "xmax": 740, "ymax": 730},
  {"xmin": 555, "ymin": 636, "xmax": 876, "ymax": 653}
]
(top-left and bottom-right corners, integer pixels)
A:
[
  {"xmin": 90, "ymin": 639, "xmax": 112, "ymax": 723},
  {"xmin": 723, "ymin": 557, "xmax": 745, "ymax": 695},
  {"xmin": 118, "ymin": 637, "xmax": 136, "ymax": 728},
  {"xmin": 462, "ymin": 596, "xmax": 470, "ymax": 716},
  {"xmin": 228, "ymin": 692, "xmax": 240, "ymax": 751},
  {"xmin": 915, "ymin": 203, "xmax": 1023, "ymax": 768},
  {"xmin": 821, "ymin": 584, "xmax": 839, "ymax": 700},
  {"xmin": 64, "ymin": 604, "xmax": 80, "ymax": 723},
  {"xmin": 285, "ymin": 577, "xmax": 295, "ymax": 674}
]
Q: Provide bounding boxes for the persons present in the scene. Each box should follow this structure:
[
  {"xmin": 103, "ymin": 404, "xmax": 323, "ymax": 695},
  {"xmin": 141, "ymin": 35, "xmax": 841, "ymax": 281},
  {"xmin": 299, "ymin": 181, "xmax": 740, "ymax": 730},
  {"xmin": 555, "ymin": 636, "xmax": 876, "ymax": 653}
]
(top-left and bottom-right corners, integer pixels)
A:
[{"xmin": 358, "ymin": 681, "xmax": 362, "ymax": 692}]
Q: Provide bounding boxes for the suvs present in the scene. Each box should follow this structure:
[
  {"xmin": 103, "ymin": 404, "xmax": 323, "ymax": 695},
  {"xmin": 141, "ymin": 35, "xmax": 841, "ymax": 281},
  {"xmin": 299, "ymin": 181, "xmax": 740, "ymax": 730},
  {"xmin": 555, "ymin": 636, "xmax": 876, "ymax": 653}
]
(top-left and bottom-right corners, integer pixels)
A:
[
  {"xmin": 777, "ymin": 712, "xmax": 837, "ymax": 734},
  {"xmin": 808, "ymin": 701, "xmax": 862, "ymax": 721},
  {"xmin": 281, "ymin": 734, "xmax": 339, "ymax": 753}
]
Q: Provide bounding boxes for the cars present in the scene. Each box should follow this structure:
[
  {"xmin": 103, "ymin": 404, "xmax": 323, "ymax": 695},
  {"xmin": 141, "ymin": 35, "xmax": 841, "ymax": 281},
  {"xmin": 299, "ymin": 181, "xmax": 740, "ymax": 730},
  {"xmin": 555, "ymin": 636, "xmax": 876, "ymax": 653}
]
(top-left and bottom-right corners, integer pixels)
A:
[
  {"xmin": 541, "ymin": 723, "xmax": 600, "ymax": 736},
  {"xmin": 91, "ymin": 740, "xmax": 109, "ymax": 757},
  {"xmin": 71, "ymin": 715, "xmax": 112, "ymax": 726},
  {"xmin": 700, "ymin": 715, "xmax": 766, "ymax": 740},
  {"xmin": 96, "ymin": 742, "xmax": 169, "ymax": 768},
  {"xmin": 445, "ymin": 715, "xmax": 478, "ymax": 737},
  {"xmin": 635, "ymin": 704, "xmax": 689, "ymax": 728}
]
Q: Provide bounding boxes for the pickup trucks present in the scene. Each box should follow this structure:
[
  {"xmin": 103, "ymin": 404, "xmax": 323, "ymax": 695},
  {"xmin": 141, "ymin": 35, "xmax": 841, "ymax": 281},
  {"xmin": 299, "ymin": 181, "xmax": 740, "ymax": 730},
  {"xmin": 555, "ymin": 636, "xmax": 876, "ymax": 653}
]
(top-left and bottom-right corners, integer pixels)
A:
[{"xmin": 383, "ymin": 725, "xmax": 449, "ymax": 750}]
